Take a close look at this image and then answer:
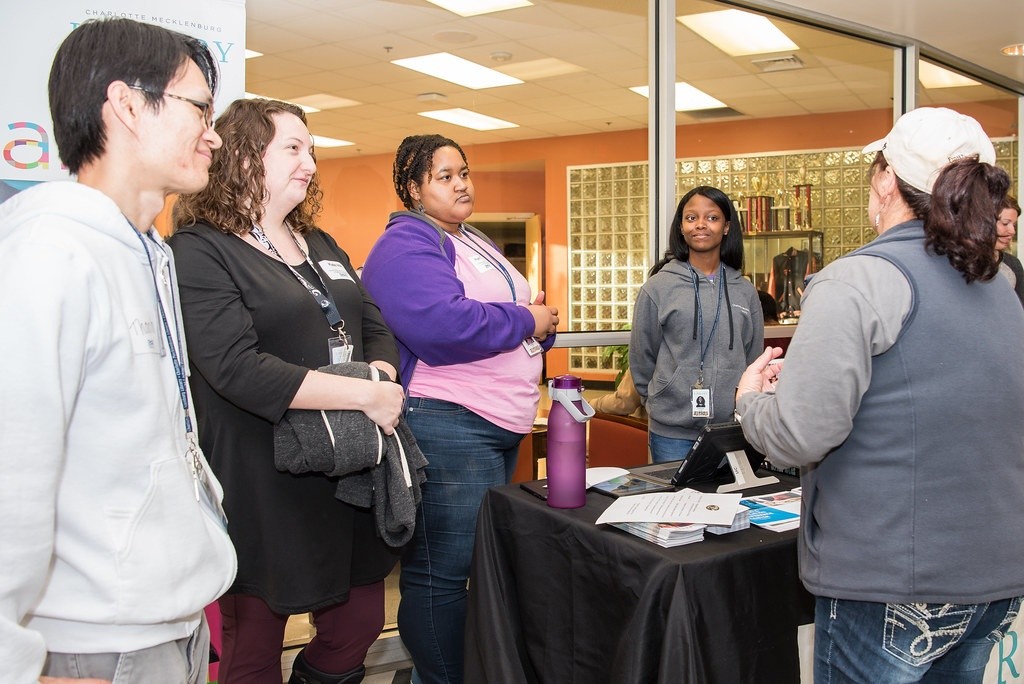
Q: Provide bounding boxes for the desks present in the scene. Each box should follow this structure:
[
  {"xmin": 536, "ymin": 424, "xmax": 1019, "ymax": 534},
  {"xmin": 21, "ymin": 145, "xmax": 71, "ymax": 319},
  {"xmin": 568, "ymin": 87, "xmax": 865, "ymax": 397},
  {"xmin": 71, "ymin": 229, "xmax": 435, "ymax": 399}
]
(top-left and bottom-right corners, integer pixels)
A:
[{"xmin": 466, "ymin": 458, "xmax": 816, "ymax": 684}]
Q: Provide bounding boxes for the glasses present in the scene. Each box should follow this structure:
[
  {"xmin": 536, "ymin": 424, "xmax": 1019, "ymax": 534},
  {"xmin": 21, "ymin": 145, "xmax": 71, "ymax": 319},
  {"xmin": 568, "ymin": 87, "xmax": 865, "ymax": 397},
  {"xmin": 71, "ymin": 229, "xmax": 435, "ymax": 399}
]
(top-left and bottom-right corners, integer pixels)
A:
[{"xmin": 126, "ymin": 82, "xmax": 214, "ymax": 129}]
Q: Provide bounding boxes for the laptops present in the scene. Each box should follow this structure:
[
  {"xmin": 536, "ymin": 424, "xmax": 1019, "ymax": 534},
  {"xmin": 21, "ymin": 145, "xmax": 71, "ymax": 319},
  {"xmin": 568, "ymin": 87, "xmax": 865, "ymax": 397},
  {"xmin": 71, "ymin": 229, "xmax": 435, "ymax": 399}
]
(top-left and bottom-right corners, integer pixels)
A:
[{"xmin": 628, "ymin": 421, "xmax": 766, "ymax": 486}]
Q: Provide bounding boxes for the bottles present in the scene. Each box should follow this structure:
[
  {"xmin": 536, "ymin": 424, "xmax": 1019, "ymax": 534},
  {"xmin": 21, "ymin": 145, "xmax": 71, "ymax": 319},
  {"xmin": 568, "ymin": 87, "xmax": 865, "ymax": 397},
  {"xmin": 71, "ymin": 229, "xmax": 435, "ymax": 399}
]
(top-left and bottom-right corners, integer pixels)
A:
[{"xmin": 547, "ymin": 375, "xmax": 595, "ymax": 509}]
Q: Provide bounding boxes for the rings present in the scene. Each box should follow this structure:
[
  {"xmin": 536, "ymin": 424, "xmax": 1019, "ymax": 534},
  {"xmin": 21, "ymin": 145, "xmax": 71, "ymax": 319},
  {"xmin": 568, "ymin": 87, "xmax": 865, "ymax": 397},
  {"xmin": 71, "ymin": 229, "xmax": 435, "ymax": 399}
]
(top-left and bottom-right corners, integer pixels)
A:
[{"xmin": 768, "ymin": 370, "xmax": 777, "ymax": 384}]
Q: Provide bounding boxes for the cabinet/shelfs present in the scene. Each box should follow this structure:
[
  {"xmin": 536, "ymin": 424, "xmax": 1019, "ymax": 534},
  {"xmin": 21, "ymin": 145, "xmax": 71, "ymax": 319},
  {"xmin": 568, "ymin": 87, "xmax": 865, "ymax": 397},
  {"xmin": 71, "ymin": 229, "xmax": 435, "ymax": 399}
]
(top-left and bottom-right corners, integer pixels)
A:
[{"xmin": 742, "ymin": 231, "xmax": 823, "ymax": 326}]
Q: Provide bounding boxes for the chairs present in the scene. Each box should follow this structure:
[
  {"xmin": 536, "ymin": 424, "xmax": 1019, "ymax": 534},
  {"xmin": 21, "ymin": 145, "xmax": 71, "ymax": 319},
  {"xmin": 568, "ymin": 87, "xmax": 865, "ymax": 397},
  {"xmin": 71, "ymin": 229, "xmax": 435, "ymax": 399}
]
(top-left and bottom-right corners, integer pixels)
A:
[{"xmin": 587, "ymin": 409, "xmax": 648, "ymax": 468}]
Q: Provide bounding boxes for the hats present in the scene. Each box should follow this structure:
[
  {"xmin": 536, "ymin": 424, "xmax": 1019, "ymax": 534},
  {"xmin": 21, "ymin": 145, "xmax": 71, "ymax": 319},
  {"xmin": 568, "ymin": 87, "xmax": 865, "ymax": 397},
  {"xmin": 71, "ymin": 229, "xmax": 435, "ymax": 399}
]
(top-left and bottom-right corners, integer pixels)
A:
[{"xmin": 860, "ymin": 106, "xmax": 997, "ymax": 194}]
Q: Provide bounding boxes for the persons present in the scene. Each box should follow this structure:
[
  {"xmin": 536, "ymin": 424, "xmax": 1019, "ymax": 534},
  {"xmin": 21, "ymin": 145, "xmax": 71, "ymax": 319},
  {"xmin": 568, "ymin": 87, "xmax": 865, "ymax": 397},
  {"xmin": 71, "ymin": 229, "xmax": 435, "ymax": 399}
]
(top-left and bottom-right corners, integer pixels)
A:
[
  {"xmin": 697, "ymin": 396, "xmax": 706, "ymax": 407},
  {"xmin": 735, "ymin": 106, "xmax": 1024, "ymax": 684},
  {"xmin": 990, "ymin": 193, "xmax": 1024, "ymax": 311},
  {"xmin": 167, "ymin": 98, "xmax": 406, "ymax": 684},
  {"xmin": 0, "ymin": 18, "xmax": 238, "ymax": 684},
  {"xmin": 360, "ymin": 134, "xmax": 560, "ymax": 684},
  {"xmin": 590, "ymin": 186, "xmax": 765, "ymax": 465}
]
